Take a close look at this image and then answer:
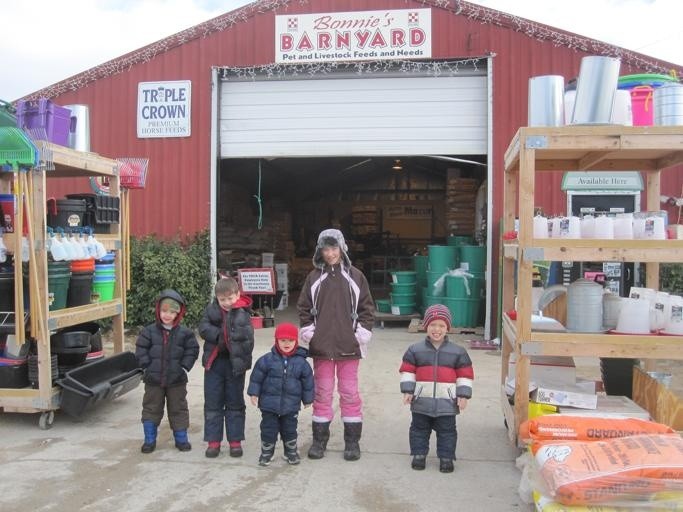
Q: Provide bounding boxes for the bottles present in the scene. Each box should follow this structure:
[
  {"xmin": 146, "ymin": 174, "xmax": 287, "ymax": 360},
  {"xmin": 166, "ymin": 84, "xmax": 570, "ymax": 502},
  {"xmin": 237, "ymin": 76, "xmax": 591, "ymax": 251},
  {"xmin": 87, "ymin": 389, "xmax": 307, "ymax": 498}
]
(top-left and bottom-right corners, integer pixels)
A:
[{"xmin": 422, "ymin": 248, "xmax": 427, "ymax": 256}]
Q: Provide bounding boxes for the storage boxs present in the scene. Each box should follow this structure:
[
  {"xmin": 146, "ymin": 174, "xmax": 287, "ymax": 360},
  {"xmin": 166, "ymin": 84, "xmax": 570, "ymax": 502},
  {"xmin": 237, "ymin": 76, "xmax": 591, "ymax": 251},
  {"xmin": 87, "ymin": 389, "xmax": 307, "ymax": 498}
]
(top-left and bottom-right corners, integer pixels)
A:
[
  {"xmin": 15, "ymin": 98, "xmax": 73, "ymax": 147},
  {"xmin": 626, "ymin": 361, "xmax": 682, "ymax": 436},
  {"xmin": 381, "ymin": 203, "xmax": 433, "ymax": 240}
]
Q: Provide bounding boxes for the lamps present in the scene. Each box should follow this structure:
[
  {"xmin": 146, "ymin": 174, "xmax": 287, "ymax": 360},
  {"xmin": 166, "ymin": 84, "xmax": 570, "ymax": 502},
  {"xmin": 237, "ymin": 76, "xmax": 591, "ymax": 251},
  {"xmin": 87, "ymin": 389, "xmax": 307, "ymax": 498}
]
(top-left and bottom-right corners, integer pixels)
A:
[{"xmin": 392, "ymin": 157, "xmax": 402, "ymax": 171}]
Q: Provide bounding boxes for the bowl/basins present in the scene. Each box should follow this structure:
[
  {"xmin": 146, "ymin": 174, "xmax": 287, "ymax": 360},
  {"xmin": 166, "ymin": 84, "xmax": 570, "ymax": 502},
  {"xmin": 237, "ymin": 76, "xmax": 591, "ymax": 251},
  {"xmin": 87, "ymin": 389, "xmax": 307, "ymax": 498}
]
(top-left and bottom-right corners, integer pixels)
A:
[
  {"xmin": 375, "ymin": 270, "xmax": 418, "ymax": 315},
  {"xmin": 0, "ymin": 345, "xmax": 57, "ymax": 389},
  {"xmin": 52, "ymin": 322, "xmax": 109, "ymax": 381}
]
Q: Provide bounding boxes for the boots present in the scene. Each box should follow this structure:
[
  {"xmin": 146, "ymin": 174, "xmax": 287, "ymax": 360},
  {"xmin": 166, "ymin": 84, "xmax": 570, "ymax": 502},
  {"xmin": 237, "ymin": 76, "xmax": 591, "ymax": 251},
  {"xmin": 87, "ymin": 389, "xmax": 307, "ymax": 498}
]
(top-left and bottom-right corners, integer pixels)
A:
[
  {"xmin": 259, "ymin": 441, "xmax": 275, "ymax": 466},
  {"xmin": 141, "ymin": 423, "xmax": 157, "ymax": 452},
  {"xmin": 343, "ymin": 422, "xmax": 362, "ymax": 460},
  {"xmin": 174, "ymin": 428, "xmax": 190, "ymax": 451},
  {"xmin": 307, "ymin": 421, "xmax": 330, "ymax": 458},
  {"xmin": 283, "ymin": 439, "xmax": 300, "ymax": 463}
]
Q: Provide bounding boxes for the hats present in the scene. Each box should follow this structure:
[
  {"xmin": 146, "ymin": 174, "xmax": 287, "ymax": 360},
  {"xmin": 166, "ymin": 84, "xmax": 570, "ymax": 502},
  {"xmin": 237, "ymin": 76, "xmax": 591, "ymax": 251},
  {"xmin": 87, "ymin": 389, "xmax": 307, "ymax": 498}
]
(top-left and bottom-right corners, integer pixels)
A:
[
  {"xmin": 276, "ymin": 323, "xmax": 298, "ymax": 356},
  {"xmin": 160, "ymin": 298, "xmax": 181, "ymax": 313},
  {"xmin": 422, "ymin": 305, "xmax": 452, "ymax": 332},
  {"xmin": 312, "ymin": 229, "xmax": 351, "ymax": 269}
]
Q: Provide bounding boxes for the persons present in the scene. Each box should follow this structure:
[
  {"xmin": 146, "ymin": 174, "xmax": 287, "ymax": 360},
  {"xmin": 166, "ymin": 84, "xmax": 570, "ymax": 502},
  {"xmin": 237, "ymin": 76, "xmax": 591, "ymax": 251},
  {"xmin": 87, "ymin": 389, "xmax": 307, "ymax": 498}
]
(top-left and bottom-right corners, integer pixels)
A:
[
  {"xmin": 133, "ymin": 289, "xmax": 200, "ymax": 454},
  {"xmin": 246, "ymin": 322, "xmax": 316, "ymax": 467},
  {"xmin": 397, "ymin": 302, "xmax": 476, "ymax": 473},
  {"xmin": 197, "ymin": 276, "xmax": 255, "ymax": 459},
  {"xmin": 294, "ymin": 228, "xmax": 377, "ymax": 463}
]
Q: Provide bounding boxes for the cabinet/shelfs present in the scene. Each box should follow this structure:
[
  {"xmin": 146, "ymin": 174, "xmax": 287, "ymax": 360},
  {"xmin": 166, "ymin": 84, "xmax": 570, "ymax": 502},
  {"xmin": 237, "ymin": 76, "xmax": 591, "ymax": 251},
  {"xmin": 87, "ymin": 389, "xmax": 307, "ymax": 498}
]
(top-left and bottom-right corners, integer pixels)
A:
[{"xmin": 370, "ymin": 253, "xmax": 410, "ymax": 294}]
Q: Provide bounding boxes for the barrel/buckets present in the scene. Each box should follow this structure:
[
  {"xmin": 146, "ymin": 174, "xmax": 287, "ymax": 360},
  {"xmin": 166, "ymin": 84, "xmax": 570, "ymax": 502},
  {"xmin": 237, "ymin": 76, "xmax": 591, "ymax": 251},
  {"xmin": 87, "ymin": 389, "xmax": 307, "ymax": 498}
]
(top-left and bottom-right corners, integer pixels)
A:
[
  {"xmin": 415, "ymin": 235, "xmax": 486, "ymax": 329},
  {"xmin": 251, "ymin": 311, "xmax": 263, "ymax": 329},
  {"xmin": 263, "ymin": 316, "xmax": 275, "ymax": 329},
  {"xmin": 0, "ymin": 192, "xmax": 118, "ymax": 389}
]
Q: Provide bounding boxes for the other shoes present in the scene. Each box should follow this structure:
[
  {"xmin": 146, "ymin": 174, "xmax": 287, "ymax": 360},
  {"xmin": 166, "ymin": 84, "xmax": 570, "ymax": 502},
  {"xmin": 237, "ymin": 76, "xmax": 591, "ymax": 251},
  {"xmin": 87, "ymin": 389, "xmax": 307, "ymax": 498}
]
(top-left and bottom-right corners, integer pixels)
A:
[
  {"xmin": 230, "ymin": 440, "xmax": 242, "ymax": 456},
  {"xmin": 412, "ymin": 454, "xmax": 425, "ymax": 469},
  {"xmin": 206, "ymin": 441, "xmax": 219, "ymax": 457},
  {"xmin": 440, "ymin": 457, "xmax": 453, "ymax": 472}
]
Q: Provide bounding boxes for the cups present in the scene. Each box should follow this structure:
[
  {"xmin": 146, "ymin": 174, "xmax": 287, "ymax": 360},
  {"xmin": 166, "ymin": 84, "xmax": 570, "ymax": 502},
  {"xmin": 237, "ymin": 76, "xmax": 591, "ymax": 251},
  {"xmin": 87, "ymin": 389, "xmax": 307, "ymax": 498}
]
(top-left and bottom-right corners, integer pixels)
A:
[
  {"xmin": 615, "ymin": 285, "xmax": 683, "ymax": 335},
  {"xmin": 516, "ymin": 211, "xmax": 667, "ymax": 241}
]
